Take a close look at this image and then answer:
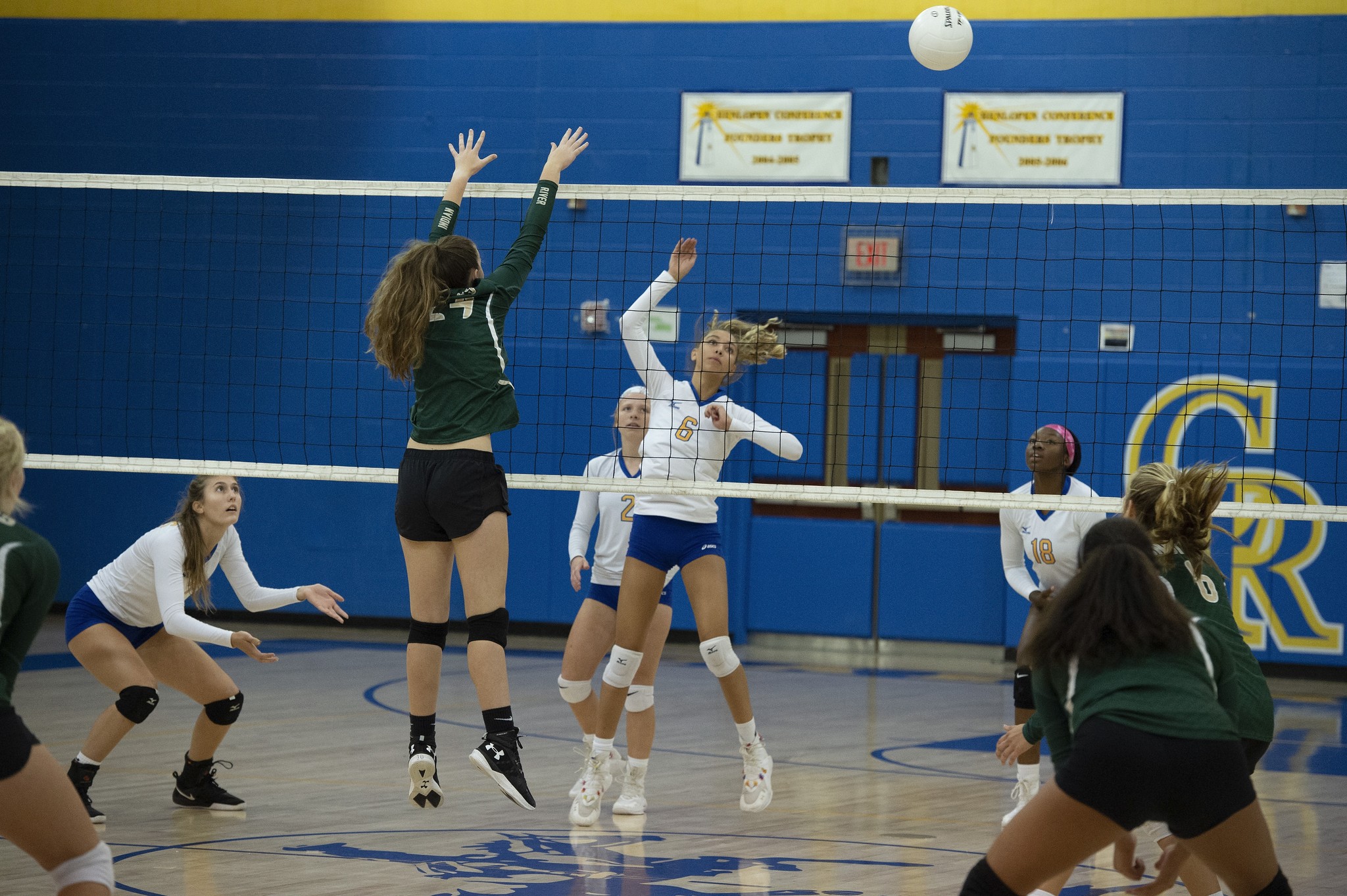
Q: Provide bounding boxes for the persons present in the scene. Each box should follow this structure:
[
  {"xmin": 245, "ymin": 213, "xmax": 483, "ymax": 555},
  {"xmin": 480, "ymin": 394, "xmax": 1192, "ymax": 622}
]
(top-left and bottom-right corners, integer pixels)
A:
[
  {"xmin": 999, "ymin": 425, "xmax": 1107, "ymax": 829},
  {"xmin": 957, "ymin": 519, "xmax": 1295, "ymax": 896},
  {"xmin": 556, "ymin": 386, "xmax": 679, "ymax": 816},
  {"xmin": 60, "ymin": 475, "xmax": 348, "ymax": 826},
  {"xmin": 360, "ymin": 125, "xmax": 591, "ymax": 810},
  {"xmin": 567, "ymin": 238, "xmax": 804, "ymax": 826},
  {"xmin": 0, "ymin": 418, "xmax": 116, "ymax": 896},
  {"xmin": 1118, "ymin": 460, "xmax": 1278, "ymax": 896}
]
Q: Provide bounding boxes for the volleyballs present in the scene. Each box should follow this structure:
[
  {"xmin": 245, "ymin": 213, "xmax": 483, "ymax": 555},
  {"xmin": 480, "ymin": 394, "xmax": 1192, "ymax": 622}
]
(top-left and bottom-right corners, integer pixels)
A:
[{"xmin": 908, "ymin": 5, "xmax": 974, "ymax": 71}]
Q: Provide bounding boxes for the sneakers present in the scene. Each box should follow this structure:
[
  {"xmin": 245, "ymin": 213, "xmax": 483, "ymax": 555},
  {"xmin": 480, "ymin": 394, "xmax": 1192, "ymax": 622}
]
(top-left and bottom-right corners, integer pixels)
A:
[
  {"xmin": 174, "ymin": 808, "xmax": 246, "ymax": 835},
  {"xmin": 737, "ymin": 859, "xmax": 771, "ymax": 895},
  {"xmin": 173, "ymin": 759, "xmax": 246, "ymax": 811},
  {"xmin": 468, "ymin": 725, "xmax": 537, "ymax": 811},
  {"xmin": 67, "ymin": 759, "xmax": 106, "ymax": 824},
  {"xmin": 613, "ymin": 761, "xmax": 647, "ymax": 814},
  {"xmin": 571, "ymin": 826, "xmax": 609, "ymax": 894},
  {"xmin": 568, "ymin": 736, "xmax": 623, "ymax": 799},
  {"xmin": 736, "ymin": 732, "xmax": 772, "ymax": 813},
  {"xmin": 570, "ymin": 748, "xmax": 613, "ymax": 826},
  {"xmin": 407, "ymin": 741, "xmax": 444, "ymax": 809},
  {"xmin": 1002, "ymin": 773, "xmax": 1040, "ymax": 826},
  {"xmin": 613, "ymin": 815, "xmax": 646, "ymax": 864}
]
